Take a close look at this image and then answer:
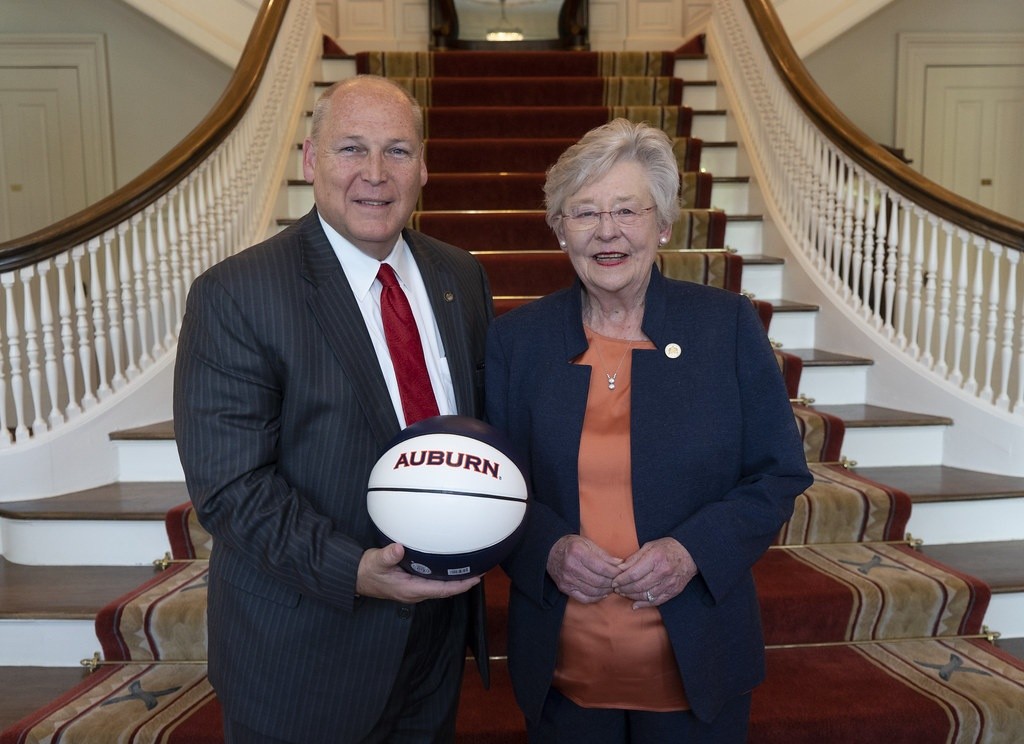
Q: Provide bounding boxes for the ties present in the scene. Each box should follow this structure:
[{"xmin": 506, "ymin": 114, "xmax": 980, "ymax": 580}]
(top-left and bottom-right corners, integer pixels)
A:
[{"xmin": 377, "ymin": 264, "xmax": 440, "ymax": 427}]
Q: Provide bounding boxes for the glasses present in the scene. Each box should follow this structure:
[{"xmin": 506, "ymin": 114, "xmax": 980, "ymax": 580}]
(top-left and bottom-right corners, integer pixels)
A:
[{"xmin": 560, "ymin": 204, "xmax": 658, "ymax": 231}]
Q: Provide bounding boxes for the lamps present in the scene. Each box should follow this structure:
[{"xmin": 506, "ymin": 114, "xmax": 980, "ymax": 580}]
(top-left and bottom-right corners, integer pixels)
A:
[{"xmin": 486, "ymin": 0, "xmax": 523, "ymax": 40}]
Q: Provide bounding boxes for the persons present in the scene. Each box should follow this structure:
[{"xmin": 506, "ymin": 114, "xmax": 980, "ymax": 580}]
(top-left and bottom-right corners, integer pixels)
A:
[
  {"xmin": 483, "ymin": 119, "xmax": 813, "ymax": 743},
  {"xmin": 174, "ymin": 73, "xmax": 495, "ymax": 744}
]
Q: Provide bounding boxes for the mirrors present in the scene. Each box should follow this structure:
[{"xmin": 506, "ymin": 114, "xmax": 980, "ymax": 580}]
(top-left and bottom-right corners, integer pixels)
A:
[{"xmin": 428, "ymin": 0, "xmax": 590, "ymax": 50}]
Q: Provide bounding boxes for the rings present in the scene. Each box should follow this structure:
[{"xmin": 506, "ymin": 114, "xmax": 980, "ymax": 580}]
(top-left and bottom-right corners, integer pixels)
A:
[{"xmin": 646, "ymin": 592, "xmax": 658, "ymax": 603}]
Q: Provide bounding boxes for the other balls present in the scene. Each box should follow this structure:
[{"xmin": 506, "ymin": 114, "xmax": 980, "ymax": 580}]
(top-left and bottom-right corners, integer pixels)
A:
[{"xmin": 362, "ymin": 414, "xmax": 533, "ymax": 582}]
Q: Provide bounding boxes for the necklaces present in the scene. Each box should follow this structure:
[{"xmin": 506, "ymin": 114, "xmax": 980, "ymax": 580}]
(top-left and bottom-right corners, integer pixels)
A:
[{"xmin": 586, "ymin": 314, "xmax": 640, "ymax": 390}]
